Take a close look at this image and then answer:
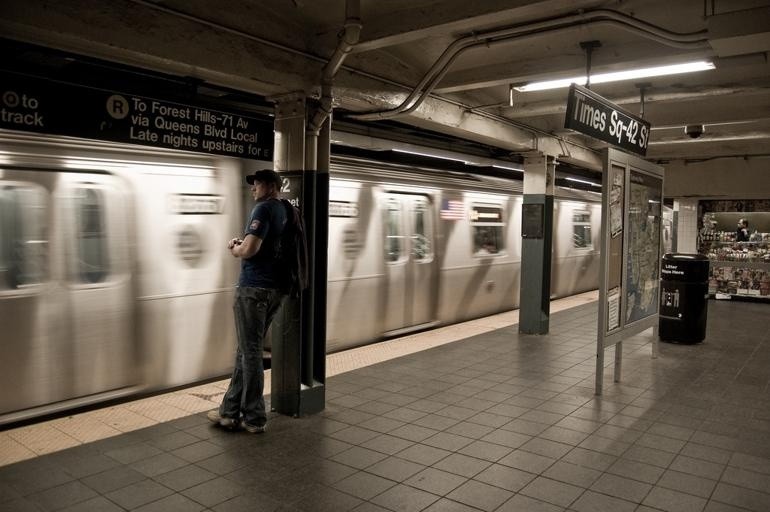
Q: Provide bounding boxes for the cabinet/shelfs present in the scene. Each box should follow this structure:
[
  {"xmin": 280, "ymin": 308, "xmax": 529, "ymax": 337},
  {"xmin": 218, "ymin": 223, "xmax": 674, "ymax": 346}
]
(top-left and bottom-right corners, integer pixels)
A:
[{"xmin": 702, "ymin": 229, "xmax": 738, "ymax": 242}]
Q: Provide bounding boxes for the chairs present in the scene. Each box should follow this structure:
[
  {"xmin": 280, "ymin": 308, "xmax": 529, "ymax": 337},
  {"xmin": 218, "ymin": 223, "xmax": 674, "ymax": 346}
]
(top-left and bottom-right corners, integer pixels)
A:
[{"xmin": 206, "ymin": 410, "xmax": 267, "ymax": 433}]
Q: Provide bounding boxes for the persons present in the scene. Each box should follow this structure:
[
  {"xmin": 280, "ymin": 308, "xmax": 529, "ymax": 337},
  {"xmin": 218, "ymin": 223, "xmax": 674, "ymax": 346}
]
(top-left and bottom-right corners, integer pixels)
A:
[
  {"xmin": 203, "ymin": 169, "xmax": 301, "ymax": 435},
  {"xmin": 735, "ymin": 217, "xmax": 750, "ymax": 241}
]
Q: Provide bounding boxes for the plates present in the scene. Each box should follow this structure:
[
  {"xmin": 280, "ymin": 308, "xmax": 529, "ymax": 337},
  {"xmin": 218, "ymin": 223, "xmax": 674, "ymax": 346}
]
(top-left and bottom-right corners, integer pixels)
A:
[{"xmin": 696, "ymin": 212, "xmax": 770, "ymax": 301}]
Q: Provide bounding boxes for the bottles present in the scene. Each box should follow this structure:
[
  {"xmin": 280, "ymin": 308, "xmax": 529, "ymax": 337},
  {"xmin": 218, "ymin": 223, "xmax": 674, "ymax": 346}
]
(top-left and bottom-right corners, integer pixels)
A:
[{"xmin": 247, "ymin": 169, "xmax": 282, "ymax": 186}]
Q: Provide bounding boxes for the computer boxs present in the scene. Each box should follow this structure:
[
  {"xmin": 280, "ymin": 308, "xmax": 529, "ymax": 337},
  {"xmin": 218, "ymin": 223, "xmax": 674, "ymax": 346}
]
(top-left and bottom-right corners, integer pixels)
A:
[{"xmin": 684, "ymin": 125, "xmax": 705, "ymax": 137}]
